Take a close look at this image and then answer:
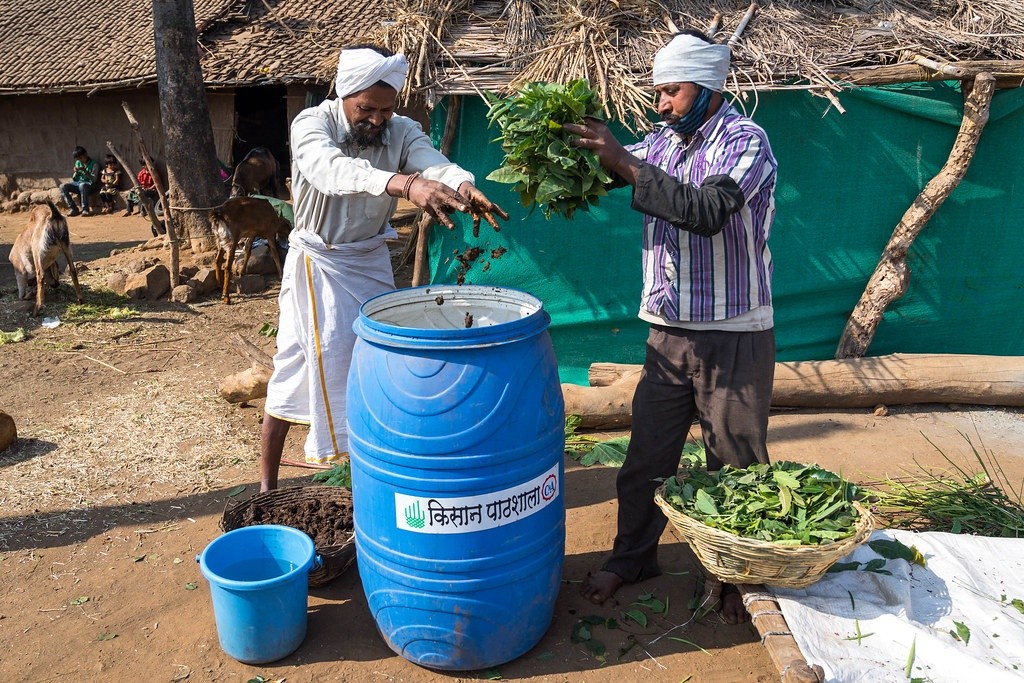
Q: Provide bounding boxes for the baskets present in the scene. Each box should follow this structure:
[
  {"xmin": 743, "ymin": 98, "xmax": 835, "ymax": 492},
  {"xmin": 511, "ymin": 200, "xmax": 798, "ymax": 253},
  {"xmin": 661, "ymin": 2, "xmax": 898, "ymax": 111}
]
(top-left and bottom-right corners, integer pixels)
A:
[{"xmin": 655, "ymin": 470, "xmax": 876, "ymax": 588}]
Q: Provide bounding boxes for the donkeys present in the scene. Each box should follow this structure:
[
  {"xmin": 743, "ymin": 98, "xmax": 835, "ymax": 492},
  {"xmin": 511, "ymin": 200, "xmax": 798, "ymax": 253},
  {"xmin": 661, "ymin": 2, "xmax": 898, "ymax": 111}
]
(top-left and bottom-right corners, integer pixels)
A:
[{"xmin": 229, "ymin": 146, "xmax": 280, "ymax": 200}]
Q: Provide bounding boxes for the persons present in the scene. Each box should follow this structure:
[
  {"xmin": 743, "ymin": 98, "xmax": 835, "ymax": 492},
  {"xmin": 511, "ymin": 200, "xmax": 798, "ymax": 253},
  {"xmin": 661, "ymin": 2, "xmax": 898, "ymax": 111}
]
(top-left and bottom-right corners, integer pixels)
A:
[
  {"xmin": 259, "ymin": 43, "xmax": 509, "ymax": 494},
  {"xmin": 59, "ymin": 146, "xmax": 100, "ymax": 216},
  {"xmin": 122, "ymin": 156, "xmax": 162, "ymax": 217},
  {"xmin": 96, "ymin": 154, "xmax": 122, "ymax": 214},
  {"xmin": 562, "ymin": 27, "xmax": 777, "ymax": 625}
]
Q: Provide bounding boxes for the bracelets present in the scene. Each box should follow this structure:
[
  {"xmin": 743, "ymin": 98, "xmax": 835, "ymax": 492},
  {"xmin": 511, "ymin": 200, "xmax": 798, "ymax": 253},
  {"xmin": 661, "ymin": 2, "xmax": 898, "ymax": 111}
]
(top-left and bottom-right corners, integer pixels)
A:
[{"xmin": 403, "ymin": 172, "xmax": 419, "ymax": 202}]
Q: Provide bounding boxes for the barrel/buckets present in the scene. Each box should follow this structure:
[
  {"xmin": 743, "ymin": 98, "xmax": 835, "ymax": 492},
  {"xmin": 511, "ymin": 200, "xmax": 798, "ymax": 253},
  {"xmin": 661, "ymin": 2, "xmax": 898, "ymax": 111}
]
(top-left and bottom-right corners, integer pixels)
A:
[
  {"xmin": 347, "ymin": 287, "xmax": 565, "ymax": 671},
  {"xmin": 196, "ymin": 524, "xmax": 323, "ymax": 664}
]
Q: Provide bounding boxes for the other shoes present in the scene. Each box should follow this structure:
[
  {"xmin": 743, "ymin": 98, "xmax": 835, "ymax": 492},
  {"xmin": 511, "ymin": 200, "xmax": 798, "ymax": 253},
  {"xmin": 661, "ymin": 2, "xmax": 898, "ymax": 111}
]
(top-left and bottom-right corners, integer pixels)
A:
[
  {"xmin": 82, "ymin": 210, "xmax": 89, "ymax": 216},
  {"xmin": 68, "ymin": 209, "xmax": 80, "ymax": 216}
]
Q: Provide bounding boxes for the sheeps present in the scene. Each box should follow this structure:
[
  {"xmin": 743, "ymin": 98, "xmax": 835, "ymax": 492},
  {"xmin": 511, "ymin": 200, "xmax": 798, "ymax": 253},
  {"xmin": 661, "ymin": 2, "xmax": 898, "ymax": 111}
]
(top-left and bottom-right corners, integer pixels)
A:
[
  {"xmin": 8, "ymin": 197, "xmax": 86, "ymax": 318},
  {"xmin": 208, "ymin": 197, "xmax": 294, "ymax": 306}
]
